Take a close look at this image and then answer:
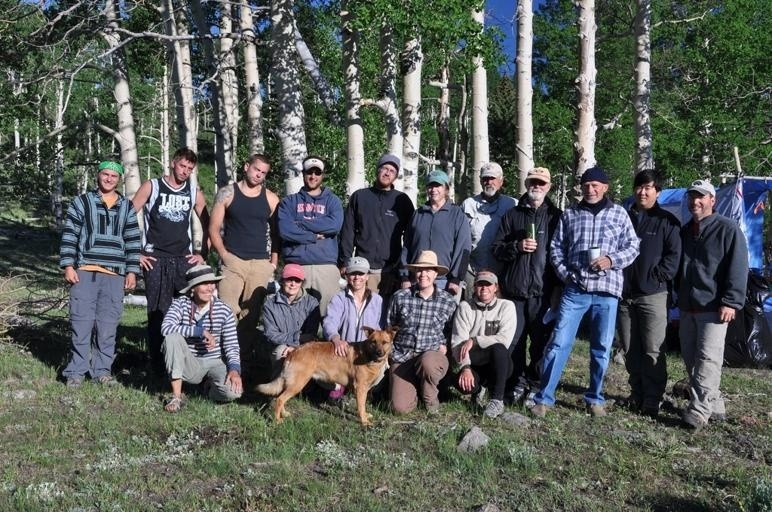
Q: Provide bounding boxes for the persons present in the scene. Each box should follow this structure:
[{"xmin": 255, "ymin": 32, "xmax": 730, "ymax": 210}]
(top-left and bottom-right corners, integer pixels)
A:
[
  {"xmin": 615, "ymin": 170, "xmax": 682, "ymax": 418},
  {"xmin": 59, "ymin": 161, "xmax": 141, "ymax": 387},
  {"xmin": 529, "ymin": 168, "xmax": 640, "ymax": 418},
  {"xmin": 668, "ymin": 180, "xmax": 749, "ymax": 428}
]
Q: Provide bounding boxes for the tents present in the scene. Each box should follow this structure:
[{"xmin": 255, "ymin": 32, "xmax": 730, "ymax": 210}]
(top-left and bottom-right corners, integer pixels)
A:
[{"xmin": 577, "ymin": 146, "xmax": 772, "ymax": 357}]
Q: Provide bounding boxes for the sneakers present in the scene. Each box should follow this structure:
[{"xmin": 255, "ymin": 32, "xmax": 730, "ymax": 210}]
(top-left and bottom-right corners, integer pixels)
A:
[
  {"xmin": 329, "ymin": 384, "xmax": 345, "ymax": 398},
  {"xmin": 485, "ymin": 399, "xmax": 504, "ymax": 419},
  {"xmin": 99, "ymin": 376, "xmax": 119, "ymax": 386},
  {"xmin": 683, "ymin": 405, "xmax": 725, "ymax": 430},
  {"xmin": 471, "ymin": 385, "xmax": 486, "ymax": 409},
  {"xmin": 524, "ymin": 392, "xmax": 536, "ymax": 409},
  {"xmin": 587, "ymin": 404, "xmax": 606, "ymax": 417},
  {"xmin": 614, "ymin": 396, "xmax": 658, "ymax": 416},
  {"xmin": 504, "ymin": 386, "xmax": 524, "ymax": 404},
  {"xmin": 67, "ymin": 377, "xmax": 82, "ymax": 387},
  {"xmin": 530, "ymin": 404, "xmax": 553, "ymax": 417}
]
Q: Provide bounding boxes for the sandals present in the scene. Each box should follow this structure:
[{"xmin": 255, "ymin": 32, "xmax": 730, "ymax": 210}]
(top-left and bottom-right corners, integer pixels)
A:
[{"xmin": 164, "ymin": 398, "xmax": 182, "ymax": 413}]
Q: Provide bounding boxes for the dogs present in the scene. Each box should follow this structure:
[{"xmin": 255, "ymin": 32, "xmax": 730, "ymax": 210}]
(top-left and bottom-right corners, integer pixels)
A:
[{"xmin": 255, "ymin": 326, "xmax": 397, "ymax": 426}]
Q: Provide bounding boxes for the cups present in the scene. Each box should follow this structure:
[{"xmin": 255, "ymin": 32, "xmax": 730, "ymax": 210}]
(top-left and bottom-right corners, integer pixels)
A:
[{"xmin": 587, "ymin": 246, "xmax": 601, "ymax": 265}]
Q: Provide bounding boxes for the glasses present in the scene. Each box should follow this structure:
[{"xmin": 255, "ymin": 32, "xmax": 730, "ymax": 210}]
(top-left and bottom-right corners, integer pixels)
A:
[{"xmin": 306, "ymin": 170, "xmax": 321, "ymax": 175}]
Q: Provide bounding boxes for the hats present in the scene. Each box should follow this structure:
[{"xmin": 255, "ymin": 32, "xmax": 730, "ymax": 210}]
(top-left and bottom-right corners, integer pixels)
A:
[
  {"xmin": 526, "ymin": 167, "xmax": 550, "ymax": 183},
  {"xmin": 474, "ymin": 272, "xmax": 498, "ymax": 285},
  {"xmin": 303, "ymin": 159, "xmax": 324, "ymax": 171},
  {"xmin": 377, "ymin": 154, "xmax": 400, "ymax": 175},
  {"xmin": 405, "ymin": 251, "xmax": 449, "ymax": 276},
  {"xmin": 346, "ymin": 257, "xmax": 370, "ymax": 274},
  {"xmin": 684, "ymin": 180, "xmax": 715, "ymax": 198},
  {"xmin": 480, "ymin": 162, "xmax": 503, "ymax": 179},
  {"xmin": 581, "ymin": 167, "xmax": 608, "ymax": 183},
  {"xmin": 426, "ymin": 170, "xmax": 449, "ymax": 190},
  {"xmin": 179, "ymin": 265, "xmax": 222, "ymax": 294},
  {"xmin": 283, "ymin": 264, "xmax": 304, "ymax": 281}
]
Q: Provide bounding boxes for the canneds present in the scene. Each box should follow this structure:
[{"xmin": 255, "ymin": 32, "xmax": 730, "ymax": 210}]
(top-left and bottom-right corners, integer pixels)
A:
[
  {"xmin": 526, "ymin": 223, "xmax": 537, "ymax": 240},
  {"xmin": 588, "ymin": 247, "xmax": 600, "ymax": 266}
]
